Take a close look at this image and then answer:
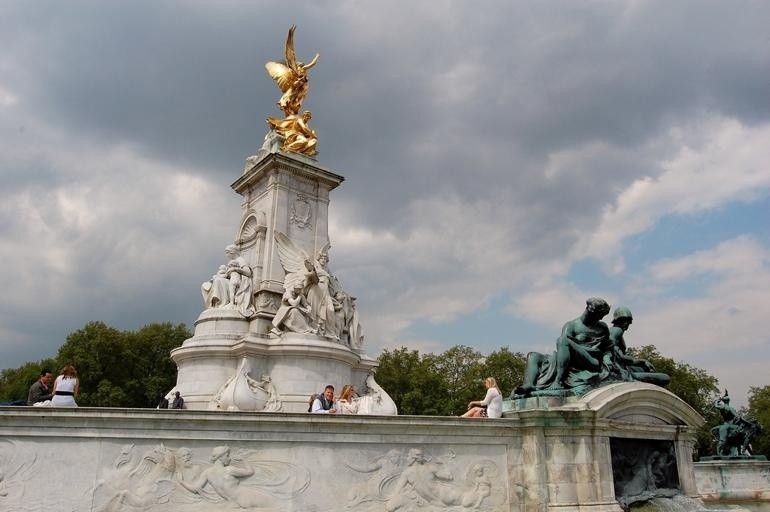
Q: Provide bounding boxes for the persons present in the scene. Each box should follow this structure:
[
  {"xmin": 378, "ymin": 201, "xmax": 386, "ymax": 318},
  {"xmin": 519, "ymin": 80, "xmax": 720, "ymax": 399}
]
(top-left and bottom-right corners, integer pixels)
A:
[
  {"xmin": 459, "ymin": 377, "xmax": 503, "ymax": 419},
  {"xmin": 608, "ymin": 306, "xmax": 672, "ymax": 385},
  {"xmin": 159, "ymin": 393, "xmax": 168, "ymax": 409},
  {"xmin": 615, "ymin": 444, "xmax": 680, "ymax": 506},
  {"xmin": 201, "ymin": 243, "xmax": 252, "ymax": 308},
  {"xmin": 714, "ymin": 394, "xmax": 743, "ymax": 425},
  {"xmin": 514, "ymin": 298, "xmax": 610, "ymax": 396},
  {"xmin": 282, "ymin": 111, "xmax": 318, "ymax": 157},
  {"xmin": 52, "ymin": 364, "xmax": 80, "ymax": 407},
  {"xmin": 271, "ymin": 254, "xmax": 364, "ymax": 348},
  {"xmin": 26, "ymin": 369, "xmax": 55, "ymax": 407},
  {"xmin": 334, "ymin": 383, "xmax": 358, "ymax": 414},
  {"xmin": 312, "ymin": 385, "xmax": 339, "ymax": 414},
  {"xmin": 224, "ymin": 260, "xmax": 240, "ymax": 306},
  {"xmin": 172, "ymin": 391, "xmax": 183, "ymax": 409},
  {"xmin": 276, "ymin": 53, "xmax": 319, "ymax": 115},
  {"xmin": 710, "ymin": 422, "xmax": 740, "ymax": 455}
]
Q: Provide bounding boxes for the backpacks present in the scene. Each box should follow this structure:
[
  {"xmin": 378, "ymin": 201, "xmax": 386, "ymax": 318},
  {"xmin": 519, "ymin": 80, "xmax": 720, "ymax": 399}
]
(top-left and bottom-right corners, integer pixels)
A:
[{"xmin": 307, "ymin": 393, "xmax": 324, "ymax": 412}]
